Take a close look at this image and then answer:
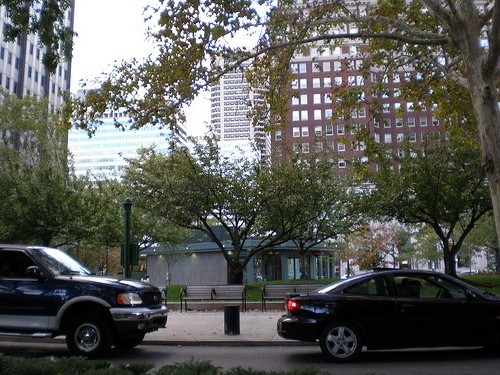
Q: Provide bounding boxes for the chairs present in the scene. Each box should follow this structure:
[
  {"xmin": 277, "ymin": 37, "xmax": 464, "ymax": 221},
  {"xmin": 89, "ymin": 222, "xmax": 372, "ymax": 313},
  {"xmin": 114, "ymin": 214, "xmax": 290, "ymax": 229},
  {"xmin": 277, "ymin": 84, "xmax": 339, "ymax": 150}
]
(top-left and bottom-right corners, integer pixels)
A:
[{"xmin": 401, "ymin": 278, "xmax": 421, "ymax": 298}]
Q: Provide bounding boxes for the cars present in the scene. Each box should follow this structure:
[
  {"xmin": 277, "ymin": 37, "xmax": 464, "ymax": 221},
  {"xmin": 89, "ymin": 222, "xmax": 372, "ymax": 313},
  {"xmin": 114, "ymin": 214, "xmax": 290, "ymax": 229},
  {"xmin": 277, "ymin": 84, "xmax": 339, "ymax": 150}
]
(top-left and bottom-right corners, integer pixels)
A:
[{"xmin": 277, "ymin": 266, "xmax": 500, "ymax": 365}]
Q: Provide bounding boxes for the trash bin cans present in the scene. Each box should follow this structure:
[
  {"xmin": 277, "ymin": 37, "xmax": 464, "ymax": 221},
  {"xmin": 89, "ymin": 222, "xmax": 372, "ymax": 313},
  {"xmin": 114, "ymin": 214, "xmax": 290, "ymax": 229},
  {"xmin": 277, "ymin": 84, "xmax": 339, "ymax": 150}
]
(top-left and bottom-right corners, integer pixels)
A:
[{"xmin": 224, "ymin": 305, "xmax": 240, "ymax": 335}]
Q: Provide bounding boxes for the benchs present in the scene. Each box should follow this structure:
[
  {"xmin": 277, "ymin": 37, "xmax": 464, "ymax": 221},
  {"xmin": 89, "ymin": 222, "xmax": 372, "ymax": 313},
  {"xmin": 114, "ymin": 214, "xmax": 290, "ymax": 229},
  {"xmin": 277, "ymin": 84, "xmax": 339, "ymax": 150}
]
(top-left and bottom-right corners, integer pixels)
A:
[
  {"xmin": 179, "ymin": 285, "xmax": 247, "ymax": 312},
  {"xmin": 262, "ymin": 284, "xmax": 326, "ymax": 312},
  {"xmin": 156, "ymin": 285, "xmax": 168, "ymax": 307}
]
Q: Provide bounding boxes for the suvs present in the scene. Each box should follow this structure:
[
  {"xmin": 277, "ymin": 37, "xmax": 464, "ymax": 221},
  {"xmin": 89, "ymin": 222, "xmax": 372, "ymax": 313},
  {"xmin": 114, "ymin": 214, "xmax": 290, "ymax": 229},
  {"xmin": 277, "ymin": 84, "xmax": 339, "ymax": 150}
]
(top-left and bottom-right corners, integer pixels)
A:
[{"xmin": 0, "ymin": 243, "xmax": 169, "ymax": 359}]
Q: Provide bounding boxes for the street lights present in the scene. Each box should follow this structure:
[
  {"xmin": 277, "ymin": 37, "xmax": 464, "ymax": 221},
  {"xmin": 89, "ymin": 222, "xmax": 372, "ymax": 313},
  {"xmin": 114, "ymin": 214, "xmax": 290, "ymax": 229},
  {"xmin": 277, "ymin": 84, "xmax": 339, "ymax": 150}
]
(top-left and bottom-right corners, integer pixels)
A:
[{"xmin": 121, "ymin": 197, "xmax": 133, "ymax": 276}]
